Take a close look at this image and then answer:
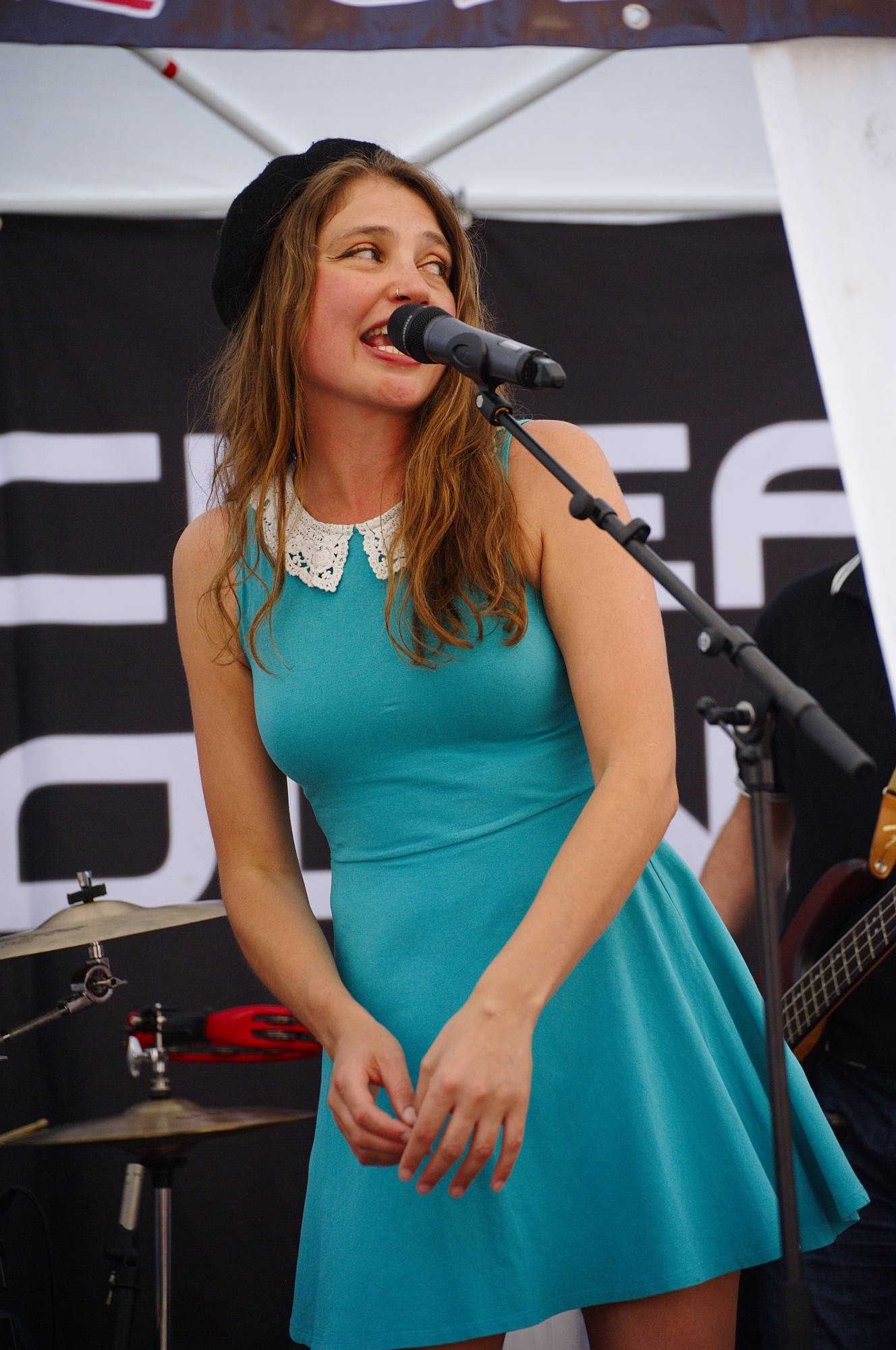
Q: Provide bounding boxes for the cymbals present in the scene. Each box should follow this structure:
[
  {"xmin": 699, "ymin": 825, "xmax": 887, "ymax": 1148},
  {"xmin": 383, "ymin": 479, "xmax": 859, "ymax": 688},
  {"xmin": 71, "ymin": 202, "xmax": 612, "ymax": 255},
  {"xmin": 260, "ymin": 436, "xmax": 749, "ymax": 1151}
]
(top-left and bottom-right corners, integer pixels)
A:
[
  {"xmin": 128, "ymin": 1002, "xmax": 321, "ymax": 1063},
  {"xmin": 5, "ymin": 1098, "xmax": 320, "ymax": 1147},
  {"xmin": 0, "ymin": 898, "xmax": 230, "ymax": 962}
]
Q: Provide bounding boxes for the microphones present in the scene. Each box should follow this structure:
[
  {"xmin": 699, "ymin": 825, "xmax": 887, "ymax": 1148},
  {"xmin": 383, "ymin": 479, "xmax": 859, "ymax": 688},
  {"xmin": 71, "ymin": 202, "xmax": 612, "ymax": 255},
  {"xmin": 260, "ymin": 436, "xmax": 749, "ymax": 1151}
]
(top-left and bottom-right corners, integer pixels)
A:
[
  {"xmin": 387, "ymin": 302, "xmax": 566, "ymax": 392},
  {"xmin": 110, "ymin": 1160, "xmax": 145, "ymax": 1350}
]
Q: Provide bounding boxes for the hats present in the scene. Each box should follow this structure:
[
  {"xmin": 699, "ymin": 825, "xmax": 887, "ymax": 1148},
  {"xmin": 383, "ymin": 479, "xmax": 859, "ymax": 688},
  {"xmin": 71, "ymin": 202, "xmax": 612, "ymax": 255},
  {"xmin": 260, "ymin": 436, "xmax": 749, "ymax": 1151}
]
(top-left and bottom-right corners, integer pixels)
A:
[{"xmin": 216, "ymin": 138, "xmax": 381, "ymax": 334}]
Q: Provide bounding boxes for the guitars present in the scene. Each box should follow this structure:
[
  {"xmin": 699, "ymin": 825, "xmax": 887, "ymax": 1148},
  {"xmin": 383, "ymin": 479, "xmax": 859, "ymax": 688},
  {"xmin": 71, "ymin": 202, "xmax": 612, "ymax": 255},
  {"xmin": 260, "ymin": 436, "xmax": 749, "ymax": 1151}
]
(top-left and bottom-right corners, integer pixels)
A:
[{"xmin": 779, "ymin": 765, "xmax": 896, "ymax": 1053}]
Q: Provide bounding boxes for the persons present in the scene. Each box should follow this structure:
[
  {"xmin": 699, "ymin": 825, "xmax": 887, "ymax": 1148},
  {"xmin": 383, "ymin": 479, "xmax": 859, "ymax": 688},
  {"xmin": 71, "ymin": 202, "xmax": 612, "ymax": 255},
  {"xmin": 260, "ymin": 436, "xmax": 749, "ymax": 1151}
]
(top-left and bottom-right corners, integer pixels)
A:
[
  {"xmin": 695, "ymin": 554, "xmax": 896, "ymax": 1350},
  {"xmin": 173, "ymin": 136, "xmax": 868, "ymax": 1350}
]
